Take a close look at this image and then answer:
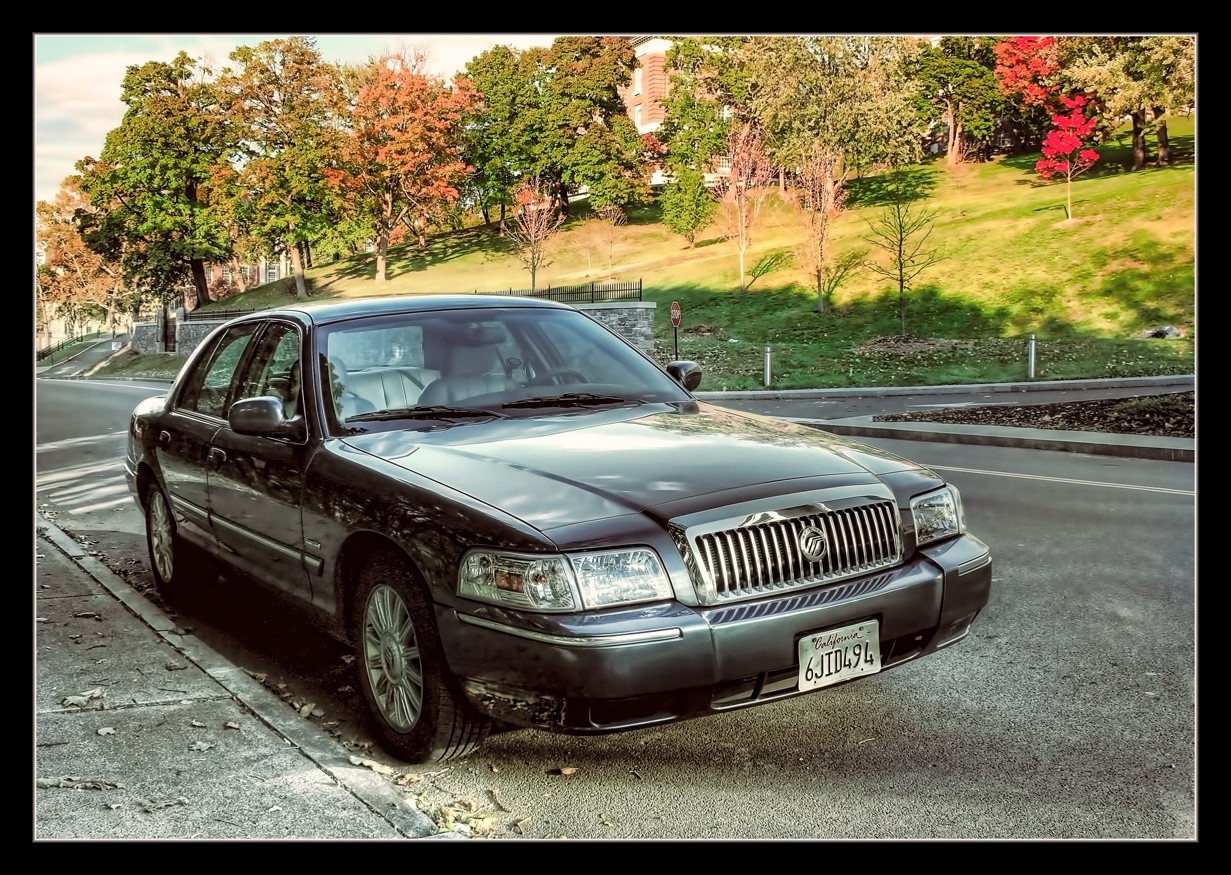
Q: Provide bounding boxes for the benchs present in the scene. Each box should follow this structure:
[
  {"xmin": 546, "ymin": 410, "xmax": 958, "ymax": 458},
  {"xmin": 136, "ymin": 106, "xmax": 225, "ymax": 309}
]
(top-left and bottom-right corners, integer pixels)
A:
[{"xmin": 201, "ymin": 369, "xmax": 441, "ymax": 415}]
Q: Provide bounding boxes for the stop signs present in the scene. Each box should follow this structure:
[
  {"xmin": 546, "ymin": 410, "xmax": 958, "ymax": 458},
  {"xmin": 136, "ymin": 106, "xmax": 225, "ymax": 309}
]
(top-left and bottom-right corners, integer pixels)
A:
[{"xmin": 669, "ymin": 301, "xmax": 682, "ymax": 329}]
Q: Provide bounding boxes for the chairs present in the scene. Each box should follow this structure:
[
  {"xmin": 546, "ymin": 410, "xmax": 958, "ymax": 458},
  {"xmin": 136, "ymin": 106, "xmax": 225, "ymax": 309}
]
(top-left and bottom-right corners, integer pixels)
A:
[
  {"xmin": 416, "ymin": 346, "xmax": 516, "ymax": 406},
  {"xmin": 282, "ymin": 356, "xmax": 378, "ymax": 419}
]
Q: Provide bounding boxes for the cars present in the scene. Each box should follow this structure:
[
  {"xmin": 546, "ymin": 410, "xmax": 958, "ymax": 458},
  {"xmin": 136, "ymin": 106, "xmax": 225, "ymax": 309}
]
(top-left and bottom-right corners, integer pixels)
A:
[{"xmin": 124, "ymin": 293, "xmax": 994, "ymax": 768}]
